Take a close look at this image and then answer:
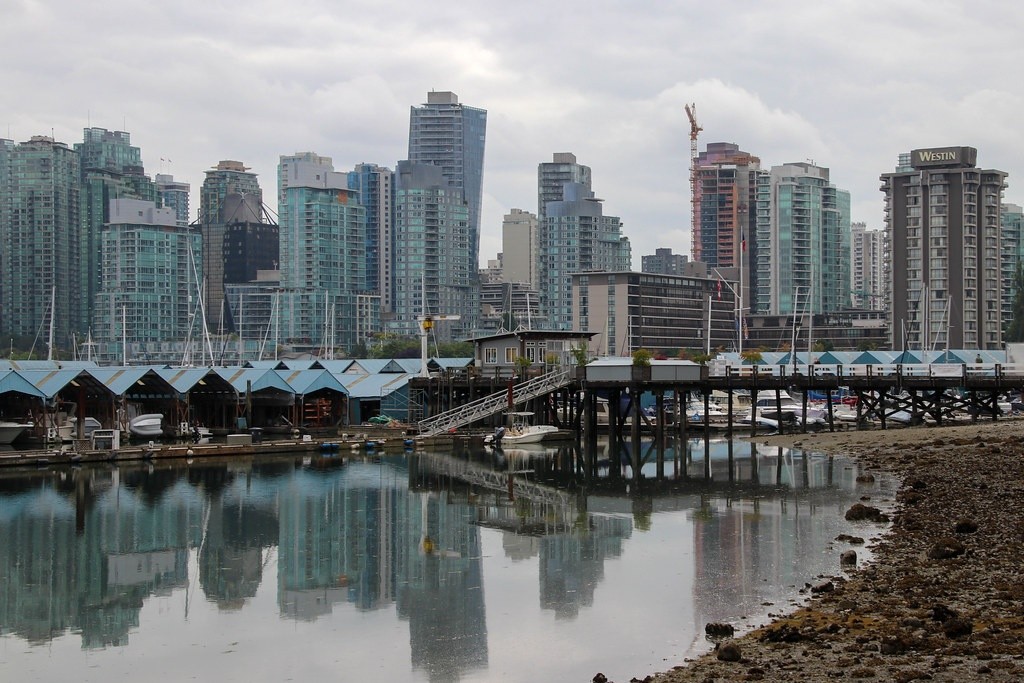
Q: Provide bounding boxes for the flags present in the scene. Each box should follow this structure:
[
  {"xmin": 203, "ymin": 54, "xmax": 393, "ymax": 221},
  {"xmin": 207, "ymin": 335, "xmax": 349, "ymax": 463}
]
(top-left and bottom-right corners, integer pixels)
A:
[
  {"xmin": 742, "ymin": 231, "xmax": 745, "ymax": 252},
  {"xmin": 717, "ymin": 278, "xmax": 722, "ymax": 301}
]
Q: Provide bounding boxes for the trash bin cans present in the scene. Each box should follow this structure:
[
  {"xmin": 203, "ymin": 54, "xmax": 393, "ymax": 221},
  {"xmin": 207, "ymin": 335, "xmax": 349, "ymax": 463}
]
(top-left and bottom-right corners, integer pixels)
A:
[
  {"xmin": 250, "ymin": 427, "xmax": 264, "ymax": 445},
  {"xmin": 666, "ymin": 412, "xmax": 673, "ymax": 425}
]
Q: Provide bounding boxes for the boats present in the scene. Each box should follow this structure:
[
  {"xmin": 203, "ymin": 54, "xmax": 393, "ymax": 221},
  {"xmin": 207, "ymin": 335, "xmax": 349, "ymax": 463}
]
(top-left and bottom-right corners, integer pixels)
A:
[
  {"xmin": 128, "ymin": 413, "xmax": 164, "ymax": 435},
  {"xmin": 0, "ymin": 418, "xmax": 34, "ymax": 445},
  {"xmin": 483, "ymin": 411, "xmax": 558, "ymax": 445},
  {"xmin": 554, "ymin": 387, "xmax": 1024, "ymax": 431},
  {"xmin": 319, "ymin": 443, "xmax": 340, "ymax": 452},
  {"xmin": 69, "ymin": 416, "xmax": 102, "ymax": 438}
]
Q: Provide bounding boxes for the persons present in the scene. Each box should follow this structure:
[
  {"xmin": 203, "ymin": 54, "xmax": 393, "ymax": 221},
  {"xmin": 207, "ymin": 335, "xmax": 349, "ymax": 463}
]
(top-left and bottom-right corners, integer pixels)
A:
[
  {"xmin": 975, "ymin": 354, "xmax": 983, "ymax": 376},
  {"xmin": 814, "ymin": 357, "xmax": 821, "ymax": 376}
]
